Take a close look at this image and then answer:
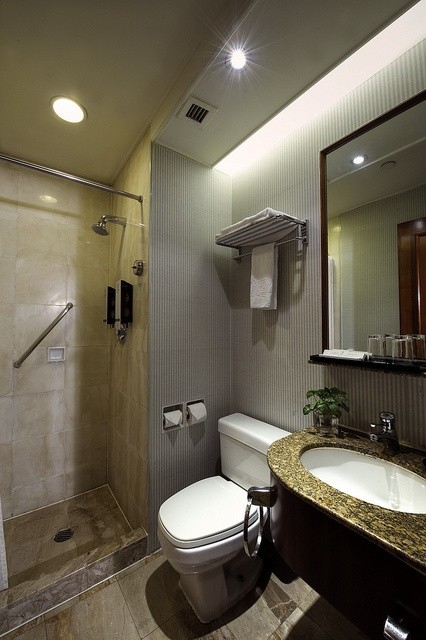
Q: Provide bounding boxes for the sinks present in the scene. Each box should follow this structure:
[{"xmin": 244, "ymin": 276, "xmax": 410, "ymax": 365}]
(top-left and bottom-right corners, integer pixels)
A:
[{"xmin": 299, "ymin": 444, "xmax": 426, "ymax": 522}]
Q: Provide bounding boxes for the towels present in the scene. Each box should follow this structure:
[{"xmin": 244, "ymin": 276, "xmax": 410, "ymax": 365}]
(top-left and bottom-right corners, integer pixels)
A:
[
  {"xmin": 214, "ymin": 209, "xmax": 298, "ymax": 245},
  {"xmin": 250, "ymin": 243, "xmax": 278, "ymax": 310}
]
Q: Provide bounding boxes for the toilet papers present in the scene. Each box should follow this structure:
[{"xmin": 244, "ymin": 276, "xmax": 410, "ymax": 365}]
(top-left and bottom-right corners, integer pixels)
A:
[
  {"xmin": 188, "ymin": 398, "xmax": 207, "ymax": 426},
  {"xmin": 163, "ymin": 407, "xmax": 184, "ymax": 433}
]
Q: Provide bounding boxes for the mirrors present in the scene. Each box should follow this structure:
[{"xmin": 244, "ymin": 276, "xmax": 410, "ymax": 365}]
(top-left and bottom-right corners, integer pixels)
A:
[{"xmin": 307, "ymin": 90, "xmax": 426, "ymax": 376}]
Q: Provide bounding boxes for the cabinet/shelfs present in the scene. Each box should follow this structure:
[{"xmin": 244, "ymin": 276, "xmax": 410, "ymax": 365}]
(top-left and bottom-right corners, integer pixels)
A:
[{"xmin": 214, "ymin": 214, "xmax": 309, "ymax": 261}]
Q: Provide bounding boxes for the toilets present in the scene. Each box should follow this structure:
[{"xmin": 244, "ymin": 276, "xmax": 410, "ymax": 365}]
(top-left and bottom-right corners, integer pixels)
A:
[{"xmin": 156, "ymin": 411, "xmax": 294, "ymax": 624}]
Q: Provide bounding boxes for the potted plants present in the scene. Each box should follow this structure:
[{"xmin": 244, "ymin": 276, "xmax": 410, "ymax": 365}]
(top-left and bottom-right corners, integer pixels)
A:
[{"xmin": 303, "ymin": 387, "xmax": 350, "ymax": 435}]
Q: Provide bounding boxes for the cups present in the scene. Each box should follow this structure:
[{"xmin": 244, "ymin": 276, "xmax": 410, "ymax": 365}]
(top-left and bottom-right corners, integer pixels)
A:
[
  {"xmin": 392, "ymin": 335, "xmax": 411, "ymax": 365},
  {"xmin": 407, "ymin": 334, "xmax": 426, "ymax": 360},
  {"xmin": 381, "ymin": 333, "xmax": 392, "ymax": 359},
  {"xmin": 367, "ymin": 335, "xmax": 384, "ymax": 359}
]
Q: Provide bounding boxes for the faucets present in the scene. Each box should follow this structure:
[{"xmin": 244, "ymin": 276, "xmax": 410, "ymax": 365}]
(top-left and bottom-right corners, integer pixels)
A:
[{"xmin": 367, "ymin": 411, "xmax": 399, "ymax": 454}]
